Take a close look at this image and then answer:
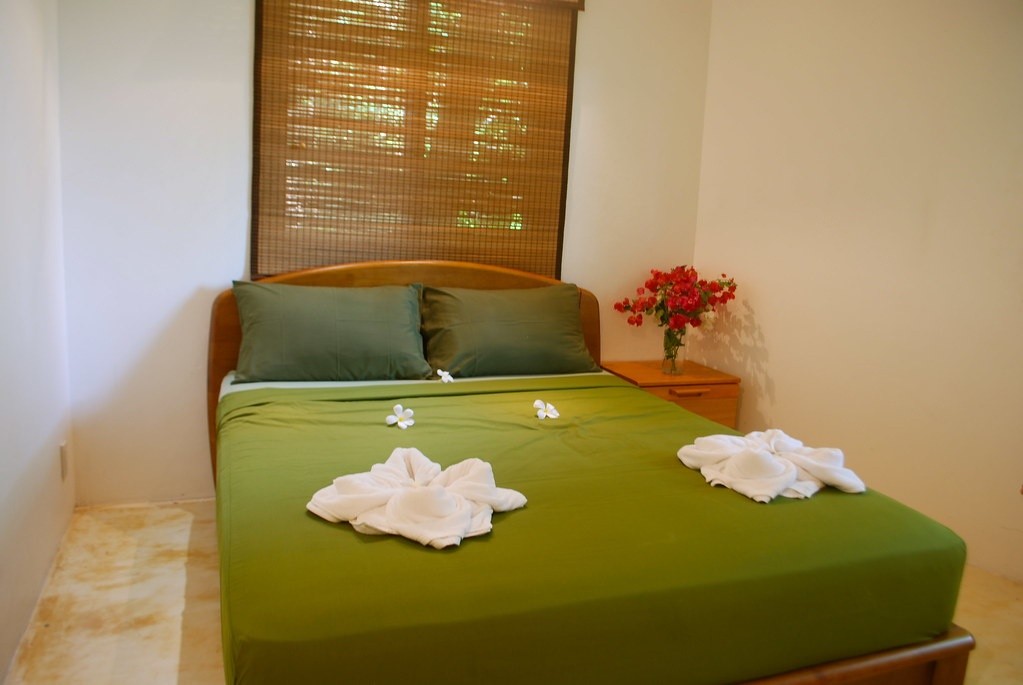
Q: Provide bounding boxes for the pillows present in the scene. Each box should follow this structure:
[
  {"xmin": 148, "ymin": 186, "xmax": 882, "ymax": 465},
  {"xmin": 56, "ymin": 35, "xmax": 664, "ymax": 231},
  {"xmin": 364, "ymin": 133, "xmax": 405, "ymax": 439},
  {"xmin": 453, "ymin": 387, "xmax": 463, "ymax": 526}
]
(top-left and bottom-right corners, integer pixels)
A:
[
  {"xmin": 231, "ymin": 280, "xmax": 432, "ymax": 382},
  {"xmin": 424, "ymin": 284, "xmax": 603, "ymax": 379}
]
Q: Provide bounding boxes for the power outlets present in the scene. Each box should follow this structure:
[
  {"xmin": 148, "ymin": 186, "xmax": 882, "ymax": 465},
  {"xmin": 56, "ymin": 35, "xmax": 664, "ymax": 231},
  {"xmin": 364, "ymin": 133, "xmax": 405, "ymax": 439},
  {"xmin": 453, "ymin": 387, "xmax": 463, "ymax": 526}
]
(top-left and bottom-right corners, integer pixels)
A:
[{"xmin": 60, "ymin": 443, "xmax": 70, "ymax": 481}]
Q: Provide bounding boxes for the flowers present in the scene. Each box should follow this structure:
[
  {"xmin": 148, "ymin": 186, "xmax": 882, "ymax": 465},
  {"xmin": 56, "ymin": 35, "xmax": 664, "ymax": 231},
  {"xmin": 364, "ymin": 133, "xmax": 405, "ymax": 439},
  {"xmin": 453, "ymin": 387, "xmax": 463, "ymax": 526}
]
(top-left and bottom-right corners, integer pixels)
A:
[
  {"xmin": 616, "ymin": 264, "xmax": 736, "ymax": 327},
  {"xmin": 437, "ymin": 368, "xmax": 453, "ymax": 383},
  {"xmin": 386, "ymin": 403, "xmax": 415, "ymax": 429},
  {"xmin": 533, "ymin": 397, "xmax": 559, "ymax": 418}
]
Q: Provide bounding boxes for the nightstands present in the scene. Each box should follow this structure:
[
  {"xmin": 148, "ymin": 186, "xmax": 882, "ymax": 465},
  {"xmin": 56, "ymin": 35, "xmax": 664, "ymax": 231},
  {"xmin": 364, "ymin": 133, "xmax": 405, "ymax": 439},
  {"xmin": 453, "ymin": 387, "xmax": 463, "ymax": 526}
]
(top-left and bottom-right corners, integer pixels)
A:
[{"xmin": 600, "ymin": 359, "xmax": 740, "ymax": 431}]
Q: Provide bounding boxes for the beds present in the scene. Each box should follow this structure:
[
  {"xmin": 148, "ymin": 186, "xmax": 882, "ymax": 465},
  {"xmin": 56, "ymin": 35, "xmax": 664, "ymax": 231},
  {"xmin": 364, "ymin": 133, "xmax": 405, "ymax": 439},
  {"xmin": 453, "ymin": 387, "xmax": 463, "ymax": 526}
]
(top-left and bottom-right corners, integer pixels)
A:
[{"xmin": 206, "ymin": 261, "xmax": 980, "ymax": 685}]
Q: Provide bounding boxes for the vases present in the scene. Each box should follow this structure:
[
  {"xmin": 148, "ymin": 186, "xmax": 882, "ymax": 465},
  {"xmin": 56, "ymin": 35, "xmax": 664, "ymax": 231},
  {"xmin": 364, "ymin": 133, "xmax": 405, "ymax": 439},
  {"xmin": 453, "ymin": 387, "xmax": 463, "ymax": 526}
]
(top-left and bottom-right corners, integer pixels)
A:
[{"xmin": 661, "ymin": 327, "xmax": 690, "ymax": 376}]
